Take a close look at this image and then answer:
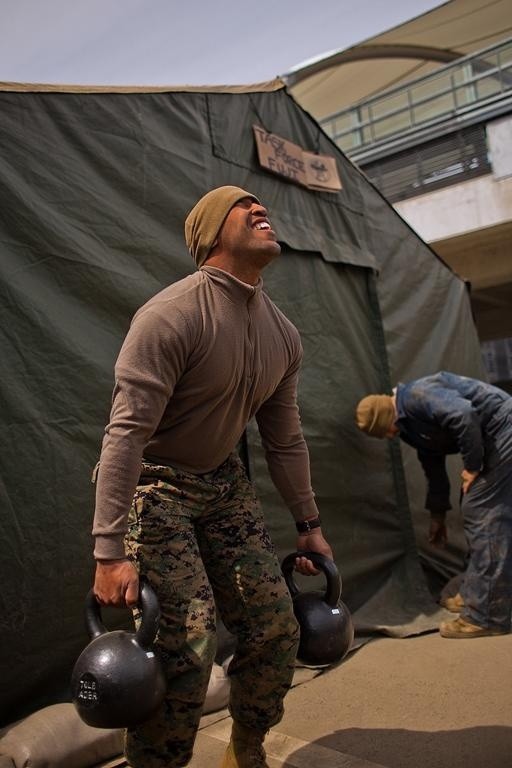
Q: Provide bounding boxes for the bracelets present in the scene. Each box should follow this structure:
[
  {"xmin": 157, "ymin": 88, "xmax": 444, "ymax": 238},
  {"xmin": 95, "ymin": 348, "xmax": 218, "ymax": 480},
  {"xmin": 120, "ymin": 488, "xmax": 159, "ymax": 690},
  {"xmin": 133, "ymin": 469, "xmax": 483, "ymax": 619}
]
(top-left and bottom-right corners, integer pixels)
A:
[{"xmin": 296, "ymin": 518, "xmax": 321, "ymax": 533}]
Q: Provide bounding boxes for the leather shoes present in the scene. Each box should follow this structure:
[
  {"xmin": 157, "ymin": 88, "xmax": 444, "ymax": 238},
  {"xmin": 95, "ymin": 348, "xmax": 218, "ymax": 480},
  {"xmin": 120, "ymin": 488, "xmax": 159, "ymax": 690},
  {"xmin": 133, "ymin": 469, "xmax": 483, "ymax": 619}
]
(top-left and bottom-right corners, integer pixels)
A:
[{"xmin": 439, "ymin": 593, "xmax": 506, "ymax": 637}]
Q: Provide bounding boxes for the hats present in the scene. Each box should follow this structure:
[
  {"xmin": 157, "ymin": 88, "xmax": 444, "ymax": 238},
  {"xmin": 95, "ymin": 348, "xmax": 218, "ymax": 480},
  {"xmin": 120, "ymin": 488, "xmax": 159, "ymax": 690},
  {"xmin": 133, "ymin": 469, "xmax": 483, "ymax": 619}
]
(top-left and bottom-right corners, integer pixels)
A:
[
  {"xmin": 185, "ymin": 186, "xmax": 260, "ymax": 269},
  {"xmin": 356, "ymin": 395, "xmax": 393, "ymax": 438}
]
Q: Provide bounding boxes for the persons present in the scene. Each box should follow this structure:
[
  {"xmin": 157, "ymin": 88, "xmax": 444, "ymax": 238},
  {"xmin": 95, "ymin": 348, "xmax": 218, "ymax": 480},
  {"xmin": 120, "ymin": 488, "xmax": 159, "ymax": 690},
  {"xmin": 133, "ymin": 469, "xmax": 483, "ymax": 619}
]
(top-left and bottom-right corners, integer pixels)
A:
[
  {"xmin": 356, "ymin": 370, "xmax": 512, "ymax": 638},
  {"xmin": 71, "ymin": 184, "xmax": 354, "ymax": 768}
]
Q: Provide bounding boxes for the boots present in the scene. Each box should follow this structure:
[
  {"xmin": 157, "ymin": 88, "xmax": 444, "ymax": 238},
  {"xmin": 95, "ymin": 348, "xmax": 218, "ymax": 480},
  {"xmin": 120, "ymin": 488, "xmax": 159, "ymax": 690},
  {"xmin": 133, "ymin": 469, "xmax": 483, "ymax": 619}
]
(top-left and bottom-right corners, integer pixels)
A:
[{"xmin": 221, "ymin": 721, "xmax": 269, "ymax": 768}]
